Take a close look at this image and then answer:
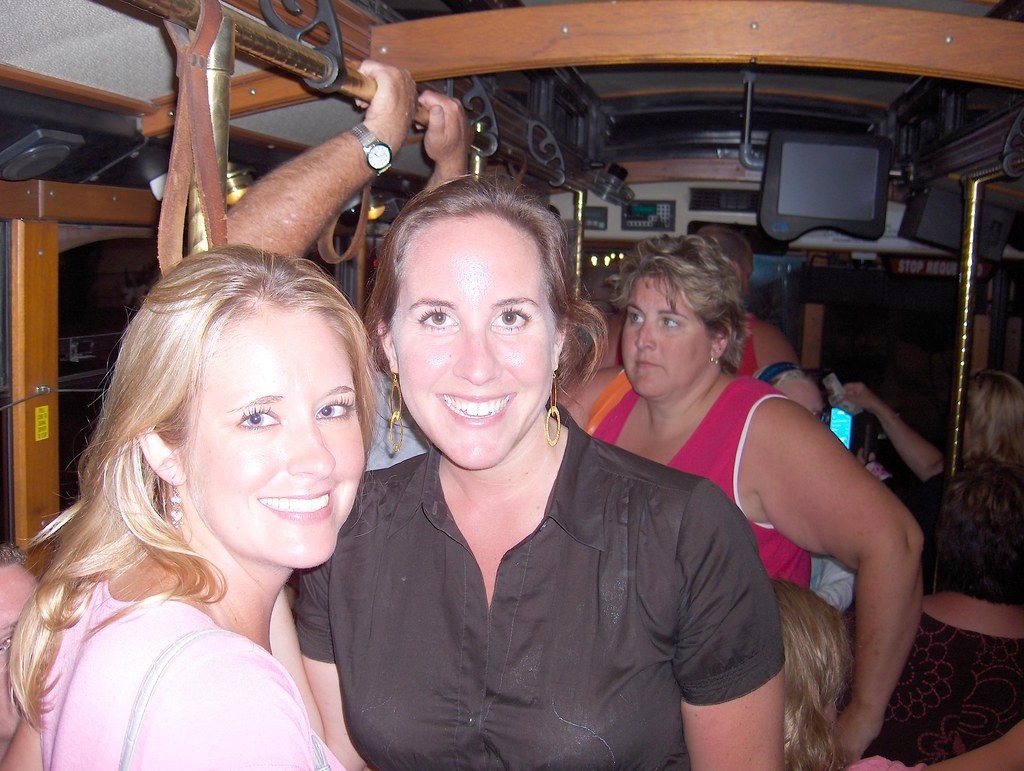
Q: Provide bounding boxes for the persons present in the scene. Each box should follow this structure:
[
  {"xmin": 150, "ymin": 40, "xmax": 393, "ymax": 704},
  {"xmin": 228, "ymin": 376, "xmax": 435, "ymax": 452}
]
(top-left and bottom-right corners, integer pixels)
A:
[
  {"xmin": 551, "ymin": 223, "xmax": 926, "ymax": 771},
  {"xmin": 768, "ymin": 575, "xmax": 1024, "ymax": 771},
  {"xmin": 293, "ymin": 165, "xmax": 785, "ymax": 771},
  {"xmin": 226, "ymin": 57, "xmax": 473, "ymax": 474},
  {"xmin": 844, "ymin": 365, "xmax": 1024, "ymax": 768},
  {"xmin": 0, "ymin": 240, "xmax": 409, "ymax": 771}
]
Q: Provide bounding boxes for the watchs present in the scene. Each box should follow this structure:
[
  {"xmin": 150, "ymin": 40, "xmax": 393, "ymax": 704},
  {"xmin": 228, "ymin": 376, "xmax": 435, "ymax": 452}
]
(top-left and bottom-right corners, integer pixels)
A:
[{"xmin": 350, "ymin": 122, "xmax": 392, "ymax": 176}]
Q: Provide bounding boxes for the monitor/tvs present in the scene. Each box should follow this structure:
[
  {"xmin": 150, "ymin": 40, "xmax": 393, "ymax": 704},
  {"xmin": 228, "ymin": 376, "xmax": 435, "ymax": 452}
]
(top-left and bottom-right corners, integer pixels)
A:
[
  {"xmin": 757, "ymin": 127, "xmax": 892, "ymax": 243},
  {"xmin": 827, "ymin": 406, "xmax": 864, "ymax": 455}
]
